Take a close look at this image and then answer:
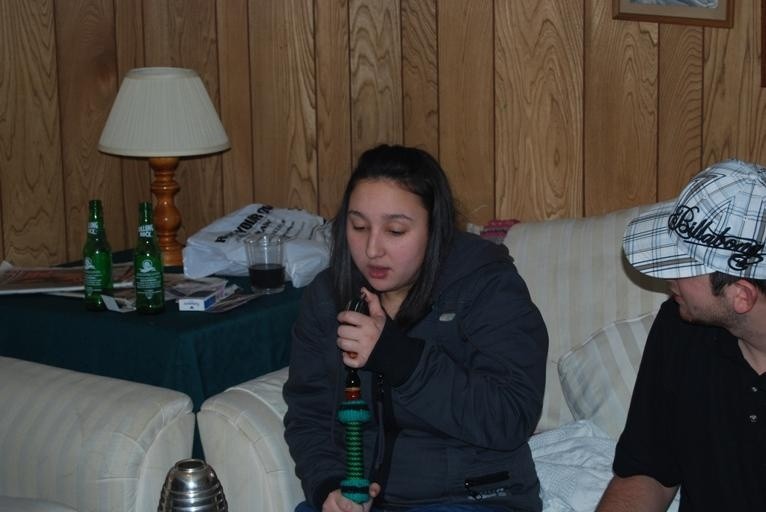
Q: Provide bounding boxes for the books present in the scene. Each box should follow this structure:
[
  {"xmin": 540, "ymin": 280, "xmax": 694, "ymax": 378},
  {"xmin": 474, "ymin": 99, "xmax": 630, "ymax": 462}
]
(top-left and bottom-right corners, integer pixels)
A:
[{"xmin": 0, "ymin": 257, "xmax": 228, "ymax": 312}]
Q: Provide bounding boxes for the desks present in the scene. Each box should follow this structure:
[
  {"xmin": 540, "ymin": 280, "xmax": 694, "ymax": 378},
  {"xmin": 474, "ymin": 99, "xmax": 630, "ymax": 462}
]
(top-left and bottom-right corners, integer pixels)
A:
[{"xmin": 5, "ymin": 251, "xmax": 316, "ymax": 450}]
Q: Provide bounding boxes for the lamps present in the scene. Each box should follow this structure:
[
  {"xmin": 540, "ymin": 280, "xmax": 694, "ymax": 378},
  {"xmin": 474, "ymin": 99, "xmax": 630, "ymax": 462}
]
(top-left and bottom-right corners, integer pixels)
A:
[{"xmin": 93, "ymin": 61, "xmax": 229, "ymax": 265}]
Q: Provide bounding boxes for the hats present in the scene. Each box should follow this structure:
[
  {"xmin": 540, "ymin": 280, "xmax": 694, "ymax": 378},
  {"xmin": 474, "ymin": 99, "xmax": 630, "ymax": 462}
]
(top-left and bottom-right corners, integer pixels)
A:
[{"xmin": 620, "ymin": 158, "xmax": 766, "ymax": 281}]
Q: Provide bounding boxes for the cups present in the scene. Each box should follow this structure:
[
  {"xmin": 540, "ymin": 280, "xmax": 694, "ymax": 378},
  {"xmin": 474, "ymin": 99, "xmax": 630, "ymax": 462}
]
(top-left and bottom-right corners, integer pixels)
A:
[{"xmin": 243, "ymin": 232, "xmax": 285, "ymax": 294}]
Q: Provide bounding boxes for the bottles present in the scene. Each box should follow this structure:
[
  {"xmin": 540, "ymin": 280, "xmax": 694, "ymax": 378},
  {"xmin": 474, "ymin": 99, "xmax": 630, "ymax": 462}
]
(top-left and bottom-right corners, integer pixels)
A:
[
  {"xmin": 157, "ymin": 456, "xmax": 229, "ymax": 512},
  {"xmin": 83, "ymin": 199, "xmax": 117, "ymax": 307},
  {"xmin": 135, "ymin": 201, "xmax": 165, "ymax": 313}
]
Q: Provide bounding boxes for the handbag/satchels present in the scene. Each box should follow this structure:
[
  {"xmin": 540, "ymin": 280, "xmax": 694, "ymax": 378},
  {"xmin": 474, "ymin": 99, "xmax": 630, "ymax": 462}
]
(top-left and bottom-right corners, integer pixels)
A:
[{"xmin": 180, "ymin": 200, "xmax": 337, "ymax": 290}]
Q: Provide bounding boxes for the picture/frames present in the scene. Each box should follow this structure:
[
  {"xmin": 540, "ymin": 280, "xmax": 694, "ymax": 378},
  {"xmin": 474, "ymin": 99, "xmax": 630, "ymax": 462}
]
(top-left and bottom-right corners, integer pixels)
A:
[{"xmin": 611, "ymin": 0, "xmax": 736, "ymax": 29}]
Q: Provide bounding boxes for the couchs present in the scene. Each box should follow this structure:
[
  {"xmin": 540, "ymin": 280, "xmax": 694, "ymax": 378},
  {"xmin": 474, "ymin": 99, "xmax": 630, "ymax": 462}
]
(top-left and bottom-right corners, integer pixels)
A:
[
  {"xmin": 205, "ymin": 198, "xmax": 676, "ymax": 512},
  {"xmin": 0, "ymin": 360, "xmax": 195, "ymax": 512}
]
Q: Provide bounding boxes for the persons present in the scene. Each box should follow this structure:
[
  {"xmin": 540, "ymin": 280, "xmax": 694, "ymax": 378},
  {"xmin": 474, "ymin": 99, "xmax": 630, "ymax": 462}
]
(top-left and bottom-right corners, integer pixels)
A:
[
  {"xmin": 280, "ymin": 141, "xmax": 551, "ymax": 512},
  {"xmin": 591, "ymin": 157, "xmax": 766, "ymax": 512}
]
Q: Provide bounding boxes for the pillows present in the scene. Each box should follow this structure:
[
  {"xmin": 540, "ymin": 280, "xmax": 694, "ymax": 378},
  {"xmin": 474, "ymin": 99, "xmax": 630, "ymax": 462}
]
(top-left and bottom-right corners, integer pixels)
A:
[{"xmin": 557, "ymin": 306, "xmax": 661, "ymax": 454}]
